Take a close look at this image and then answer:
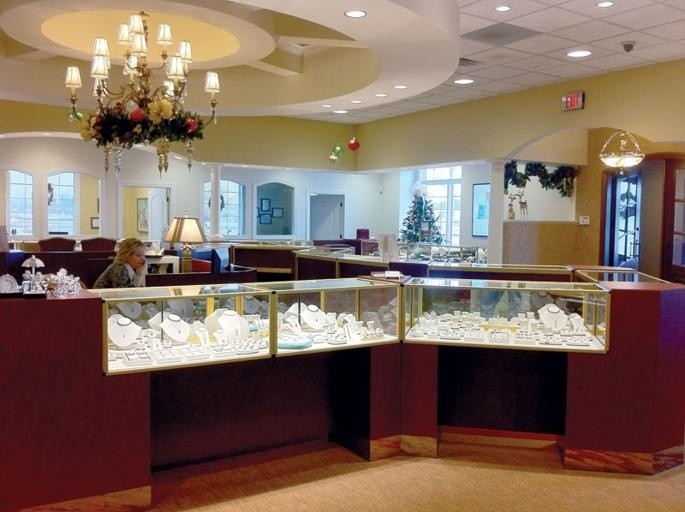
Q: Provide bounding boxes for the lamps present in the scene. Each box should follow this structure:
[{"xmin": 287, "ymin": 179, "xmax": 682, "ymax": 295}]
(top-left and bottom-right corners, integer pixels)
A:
[
  {"xmin": 164, "ymin": 211, "xmax": 208, "ymax": 272},
  {"xmin": 329, "ymin": 132, "xmax": 360, "ymax": 160},
  {"xmin": 65, "ymin": 11, "xmax": 220, "ymax": 179},
  {"xmin": 599, "ymin": 130, "xmax": 645, "ymax": 176}
]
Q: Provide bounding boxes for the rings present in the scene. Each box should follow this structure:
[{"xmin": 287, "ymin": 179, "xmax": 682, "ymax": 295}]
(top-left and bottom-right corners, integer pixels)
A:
[
  {"xmin": 414, "ymin": 303, "xmax": 588, "ymax": 345},
  {"xmin": 114, "ymin": 304, "xmax": 380, "ymax": 362}
]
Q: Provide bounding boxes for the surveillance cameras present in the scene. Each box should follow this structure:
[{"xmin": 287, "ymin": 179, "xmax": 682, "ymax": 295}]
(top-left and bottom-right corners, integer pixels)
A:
[{"xmin": 620, "ymin": 40, "xmax": 636, "ymax": 52}]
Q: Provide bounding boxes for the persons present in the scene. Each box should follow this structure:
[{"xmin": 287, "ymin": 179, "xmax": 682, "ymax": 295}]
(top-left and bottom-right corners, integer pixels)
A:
[{"xmin": 93, "ymin": 237, "xmax": 149, "ymax": 289}]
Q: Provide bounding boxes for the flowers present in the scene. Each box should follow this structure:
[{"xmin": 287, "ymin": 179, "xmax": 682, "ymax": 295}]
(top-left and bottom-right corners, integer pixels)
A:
[{"xmin": 68, "ymin": 99, "xmax": 204, "ymax": 149}]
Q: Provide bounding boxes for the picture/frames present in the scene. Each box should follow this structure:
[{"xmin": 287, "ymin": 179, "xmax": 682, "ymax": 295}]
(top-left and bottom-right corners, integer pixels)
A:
[
  {"xmin": 91, "ymin": 217, "xmax": 99, "ymax": 228},
  {"xmin": 137, "ymin": 197, "xmax": 149, "ymax": 233},
  {"xmin": 257, "ymin": 198, "xmax": 284, "ymax": 224},
  {"xmin": 472, "ymin": 183, "xmax": 490, "ymax": 236}
]
